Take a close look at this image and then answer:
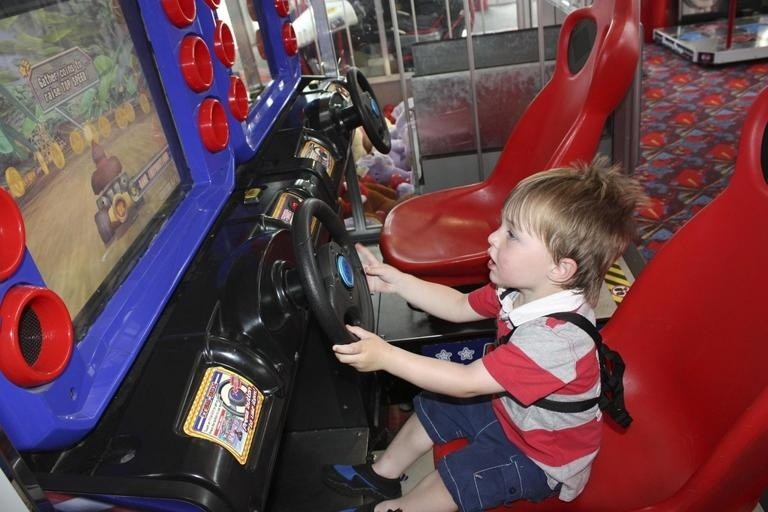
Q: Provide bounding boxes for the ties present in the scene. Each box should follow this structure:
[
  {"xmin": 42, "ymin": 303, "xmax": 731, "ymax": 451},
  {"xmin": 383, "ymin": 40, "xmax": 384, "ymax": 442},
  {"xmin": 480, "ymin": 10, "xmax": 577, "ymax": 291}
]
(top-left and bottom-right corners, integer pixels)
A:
[
  {"xmin": 0, "ymin": 0, "xmax": 182, "ymax": 322},
  {"xmin": 216, "ymin": 0, "xmax": 273, "ymax": 109}
]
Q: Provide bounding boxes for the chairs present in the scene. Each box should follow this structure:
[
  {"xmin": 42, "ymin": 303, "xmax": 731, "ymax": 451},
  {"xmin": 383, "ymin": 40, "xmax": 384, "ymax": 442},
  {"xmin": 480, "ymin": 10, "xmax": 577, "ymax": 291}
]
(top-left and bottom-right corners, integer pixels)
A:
[
  {"xmin": 340, "ymin": 499, "xmax": 403, "ymax": 512},
  {"xmin": 432, "ymin": 78, "xmax": 768, "ymax": 511},
  {"xmin": 320, "ymin": 455, "xmax": 408, "ymax": 502},
  {"xmin": 380, "ymin": 1, "xmax": 640, "ymax": 311}
]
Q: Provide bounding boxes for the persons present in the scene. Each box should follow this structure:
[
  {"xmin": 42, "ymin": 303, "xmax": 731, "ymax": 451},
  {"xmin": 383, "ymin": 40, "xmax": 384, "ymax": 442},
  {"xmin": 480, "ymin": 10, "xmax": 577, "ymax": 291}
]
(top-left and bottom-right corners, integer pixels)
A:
[{"xmin": 319, "ymin": 151, "xmax": 654, "ymax": 512}]
